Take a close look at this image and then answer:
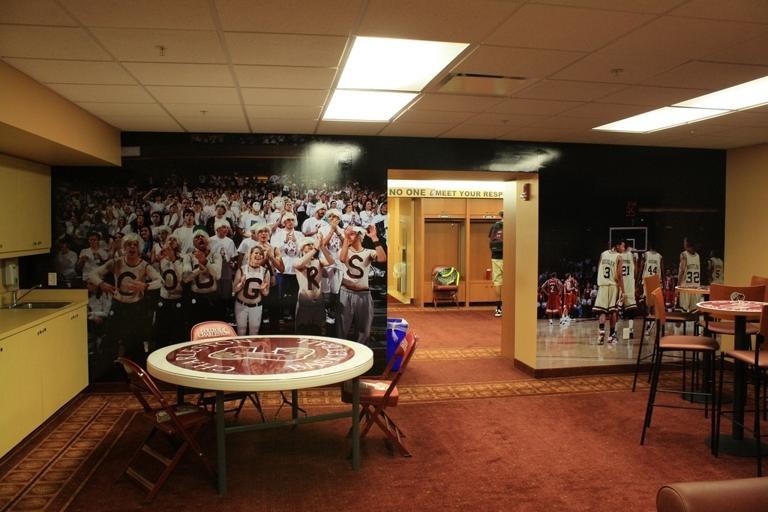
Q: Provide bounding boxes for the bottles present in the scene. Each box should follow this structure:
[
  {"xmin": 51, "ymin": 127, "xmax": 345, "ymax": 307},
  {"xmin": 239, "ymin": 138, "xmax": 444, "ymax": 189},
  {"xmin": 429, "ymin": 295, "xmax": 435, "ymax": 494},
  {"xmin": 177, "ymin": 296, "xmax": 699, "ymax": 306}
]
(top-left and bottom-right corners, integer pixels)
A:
[{"xmin": 486, "ymin": 269, "xmax": 492, "ymax": 280}]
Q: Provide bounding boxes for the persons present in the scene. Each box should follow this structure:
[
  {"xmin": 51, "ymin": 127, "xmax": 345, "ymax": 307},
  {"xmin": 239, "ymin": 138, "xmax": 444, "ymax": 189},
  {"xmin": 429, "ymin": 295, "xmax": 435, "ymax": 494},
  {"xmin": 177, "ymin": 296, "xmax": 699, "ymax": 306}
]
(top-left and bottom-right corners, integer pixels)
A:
[
  {"xmin": 488, "ymin": 211, "xmax": 503, "ymax": 317},
  {"xmin": 52, "ymin": 170, "xmax": 388, "ymax": 386},
  {"xmin": 537, "ymin": 234, "xmax": 723, "ymax": 345}
]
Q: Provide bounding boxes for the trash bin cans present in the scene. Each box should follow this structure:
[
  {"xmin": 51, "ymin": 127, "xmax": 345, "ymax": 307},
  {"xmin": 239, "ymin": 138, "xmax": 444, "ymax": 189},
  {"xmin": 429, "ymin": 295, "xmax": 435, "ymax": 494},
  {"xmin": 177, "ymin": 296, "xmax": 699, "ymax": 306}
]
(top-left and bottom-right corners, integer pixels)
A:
[{"xmin": 387, "ymin": 318, "xmax": 409, "ymax": 372}]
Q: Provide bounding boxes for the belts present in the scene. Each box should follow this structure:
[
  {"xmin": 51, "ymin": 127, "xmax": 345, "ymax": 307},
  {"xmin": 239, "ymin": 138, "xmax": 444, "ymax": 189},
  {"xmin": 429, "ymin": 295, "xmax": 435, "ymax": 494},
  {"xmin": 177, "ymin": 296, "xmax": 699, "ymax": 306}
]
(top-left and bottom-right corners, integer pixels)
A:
[{"xmin": 237, "ymin": 298, "xmax": 263, "ymax": 308}]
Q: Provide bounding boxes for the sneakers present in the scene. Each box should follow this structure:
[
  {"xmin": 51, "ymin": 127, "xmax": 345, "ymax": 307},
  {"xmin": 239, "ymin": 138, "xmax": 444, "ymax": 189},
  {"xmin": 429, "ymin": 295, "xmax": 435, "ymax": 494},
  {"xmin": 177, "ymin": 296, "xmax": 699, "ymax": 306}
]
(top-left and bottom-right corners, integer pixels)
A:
[
  {"xmin": 597, "ymin": 329, "xmax": 606, "ymax": 345},
  {"xmin": 494, "ymin": 308, "xmax": 502, "ymax": 317},
  {"xmin": 608, "ymin": 333, "xmax": 619, "ymax": 344}
]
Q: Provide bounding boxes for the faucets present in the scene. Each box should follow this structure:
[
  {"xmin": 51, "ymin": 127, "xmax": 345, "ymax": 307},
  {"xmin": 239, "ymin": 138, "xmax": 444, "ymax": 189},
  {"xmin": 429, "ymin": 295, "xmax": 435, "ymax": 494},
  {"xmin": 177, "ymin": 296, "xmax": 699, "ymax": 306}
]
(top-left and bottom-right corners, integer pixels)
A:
[{"xmin": 11, "ymin": 284, "xmax": 43, "ymax": 306}]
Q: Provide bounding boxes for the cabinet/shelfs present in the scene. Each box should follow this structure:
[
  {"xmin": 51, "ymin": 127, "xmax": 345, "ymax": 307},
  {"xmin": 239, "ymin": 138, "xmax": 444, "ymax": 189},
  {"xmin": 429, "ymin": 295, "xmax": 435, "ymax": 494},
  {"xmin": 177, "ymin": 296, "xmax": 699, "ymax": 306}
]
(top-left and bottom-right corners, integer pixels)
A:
[
  {"xmin": 0, "ymin": 304, "xmax": 89, "ymax": 461},
  {"xmin": 0, "ymin": 153, "xmax": 52, "ymax": 254}
]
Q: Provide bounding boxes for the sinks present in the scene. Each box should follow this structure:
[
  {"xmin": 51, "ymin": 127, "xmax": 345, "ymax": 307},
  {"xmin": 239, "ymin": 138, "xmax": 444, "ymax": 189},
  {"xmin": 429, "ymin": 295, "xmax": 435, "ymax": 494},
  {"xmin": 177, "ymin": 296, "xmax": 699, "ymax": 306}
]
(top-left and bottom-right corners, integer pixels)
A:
[{"xmin": 16, "ymin": 301, "xmax": 72, "ymax": 309}]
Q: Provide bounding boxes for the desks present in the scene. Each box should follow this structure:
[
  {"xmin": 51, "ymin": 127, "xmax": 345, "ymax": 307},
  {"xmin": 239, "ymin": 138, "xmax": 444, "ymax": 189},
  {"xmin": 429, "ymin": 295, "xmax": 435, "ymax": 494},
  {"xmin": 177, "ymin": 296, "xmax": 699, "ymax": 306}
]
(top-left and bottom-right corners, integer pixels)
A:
[{"xmin": 147, "ymin": 335, "xmax": 374, "ymax": 497}]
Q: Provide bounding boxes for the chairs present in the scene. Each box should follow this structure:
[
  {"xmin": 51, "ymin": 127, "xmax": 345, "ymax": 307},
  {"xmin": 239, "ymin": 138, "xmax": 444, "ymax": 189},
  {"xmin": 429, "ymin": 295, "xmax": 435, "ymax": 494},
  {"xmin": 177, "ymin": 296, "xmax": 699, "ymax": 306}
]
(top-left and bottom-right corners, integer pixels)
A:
[
  {"xmin": 632, "ymin": 274, "xmax": 768, "ymax": 477},
  {"xmin": 191, "ymin": 321, "xmax": 265, "ymax": 429},
  {"xmin": 432, "ymin": 265, "xmax": 461, "ymax": 309},
  {"xmin": 116, "ymin": 356, "xmax": 218, "ymax": 503},
  {"xmin": 341, "ymin": 329, "xmax": 420, "ymax": 458}
]
